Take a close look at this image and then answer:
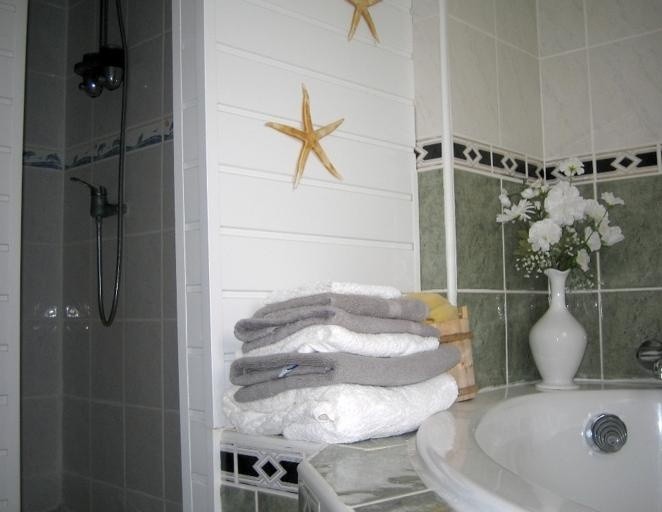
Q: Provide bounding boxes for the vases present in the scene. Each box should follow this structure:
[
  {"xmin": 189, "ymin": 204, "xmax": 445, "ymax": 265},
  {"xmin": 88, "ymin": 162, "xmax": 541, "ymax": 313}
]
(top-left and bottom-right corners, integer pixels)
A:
[{"xmin": 528, "ymin": 266, "xmax": 589, "ymax": 393}]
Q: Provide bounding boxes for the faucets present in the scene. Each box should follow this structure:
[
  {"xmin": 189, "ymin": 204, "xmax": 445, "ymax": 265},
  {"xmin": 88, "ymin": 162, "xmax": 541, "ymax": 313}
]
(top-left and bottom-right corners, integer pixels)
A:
[{"xmin": 636, "ymin": 339, "xmax": 661, "ymax": 377}]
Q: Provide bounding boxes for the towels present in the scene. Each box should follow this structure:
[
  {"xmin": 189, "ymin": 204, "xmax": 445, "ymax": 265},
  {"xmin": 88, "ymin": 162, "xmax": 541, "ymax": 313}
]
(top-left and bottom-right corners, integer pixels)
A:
[{"xmin": 266, "ymin": 282, "xmax": 401, "ymax": 299}]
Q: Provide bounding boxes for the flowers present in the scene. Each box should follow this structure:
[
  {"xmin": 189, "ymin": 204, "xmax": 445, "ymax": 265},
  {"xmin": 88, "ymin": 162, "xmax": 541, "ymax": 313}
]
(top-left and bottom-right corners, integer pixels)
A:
[{"xmin": 495, "ymin": 157, "xmax": 626, "ymax": 314}]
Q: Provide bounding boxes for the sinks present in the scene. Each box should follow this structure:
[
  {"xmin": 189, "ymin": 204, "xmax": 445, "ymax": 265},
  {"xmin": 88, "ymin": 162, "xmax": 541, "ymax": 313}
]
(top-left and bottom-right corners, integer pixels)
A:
[{"xmin": 416, "ymin": 381, "xmax": 662, "ymax": 512}]
